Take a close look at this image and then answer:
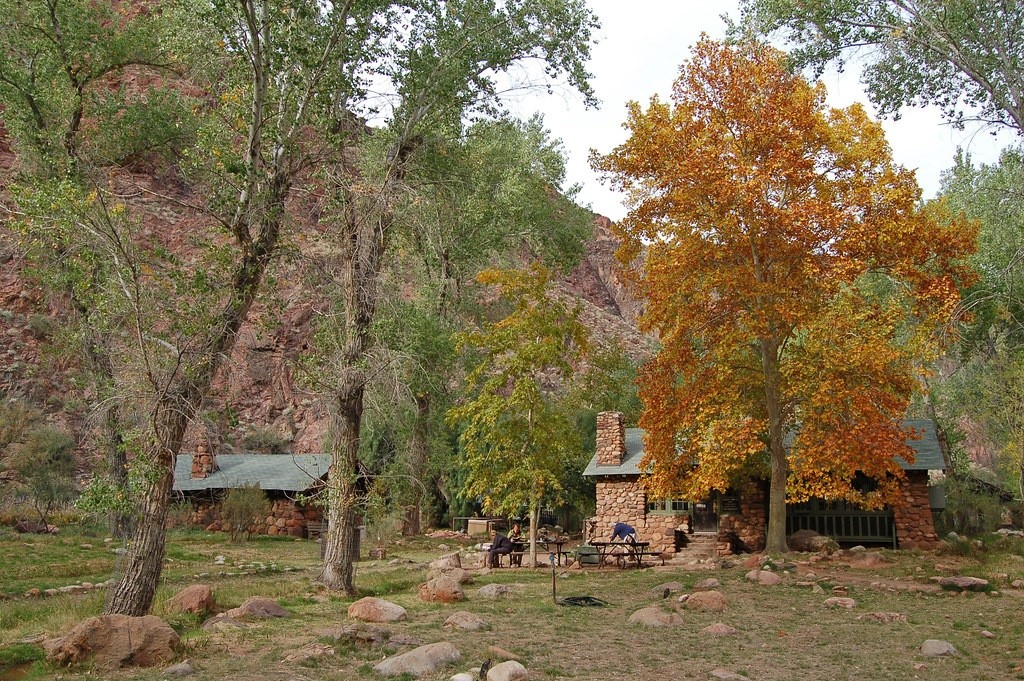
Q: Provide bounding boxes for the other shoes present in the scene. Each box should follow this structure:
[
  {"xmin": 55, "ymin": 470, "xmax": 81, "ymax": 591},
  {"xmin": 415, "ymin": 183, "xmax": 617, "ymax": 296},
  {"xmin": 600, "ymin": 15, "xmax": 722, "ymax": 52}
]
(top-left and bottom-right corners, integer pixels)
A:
[{"xmin": 487, "ymin": 563, "xmax": 492, "ymax": 569}]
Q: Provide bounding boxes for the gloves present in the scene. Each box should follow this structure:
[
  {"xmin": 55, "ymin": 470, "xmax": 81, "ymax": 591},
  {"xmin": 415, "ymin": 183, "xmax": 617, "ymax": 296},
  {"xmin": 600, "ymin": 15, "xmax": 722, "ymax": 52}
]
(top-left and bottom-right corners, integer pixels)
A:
[
  {"xmin": 488, "ymin": 549, "xmax": 491, "ymax": 552},
  {"xmin": 490, "ymin": 545, "xmax": 493, "ymax": 548}
]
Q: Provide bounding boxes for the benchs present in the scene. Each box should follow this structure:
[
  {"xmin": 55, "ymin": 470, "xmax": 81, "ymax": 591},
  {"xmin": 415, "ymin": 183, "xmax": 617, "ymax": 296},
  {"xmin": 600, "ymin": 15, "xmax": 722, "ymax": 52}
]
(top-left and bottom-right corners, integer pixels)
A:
[
  {"xmin": 613, "ymin": 551, "xmax": 665, "ymax": 570},
  {"xmin": 511, "ymin": 551, "xmax": 571, "ymax": 566},
  {"xmin": 578, "ymin": 552, "xmax": 624, "ymax": 568}
]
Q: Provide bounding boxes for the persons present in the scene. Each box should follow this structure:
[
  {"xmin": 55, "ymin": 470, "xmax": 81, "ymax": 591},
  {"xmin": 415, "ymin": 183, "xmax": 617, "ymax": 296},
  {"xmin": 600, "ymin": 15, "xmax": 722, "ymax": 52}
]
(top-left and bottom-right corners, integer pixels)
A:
[
  {"xmin": 506, "ymin": 523, "xmax": 527, "ymax": 567},
  {"xmin": 486, "ymin": 530, "xmax": 513, "ymax": 569},
  {"xmin": 608, "ymin": 523, "xmax": 639, "ymax": 563}
]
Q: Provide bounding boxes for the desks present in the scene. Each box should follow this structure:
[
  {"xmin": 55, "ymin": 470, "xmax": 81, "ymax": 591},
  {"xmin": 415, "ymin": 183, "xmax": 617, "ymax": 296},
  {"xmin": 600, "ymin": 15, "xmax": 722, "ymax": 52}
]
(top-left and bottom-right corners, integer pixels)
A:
[
  {"xmin": 591, "ymin": 542, "xmax": 649, "ymax": 569},
  {"xmin": 510, "ymin": 541, "xmax": 566, "ymax": 567}
]
